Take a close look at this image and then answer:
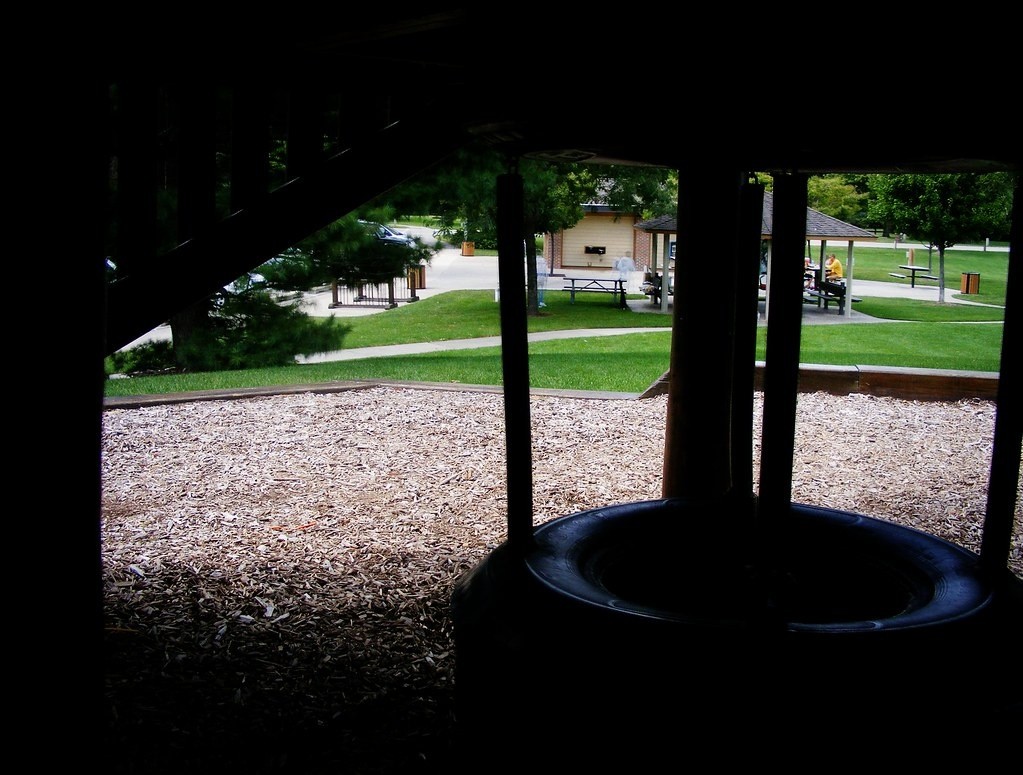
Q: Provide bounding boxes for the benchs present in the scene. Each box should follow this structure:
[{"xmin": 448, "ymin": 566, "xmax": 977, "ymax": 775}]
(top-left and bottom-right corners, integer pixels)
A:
[
  {"xmin": 561, "ymin": 286, "xmax": 626, "ymax": 294},
  {"xmin": 920, "ymin": 274, "xmax": 939, "ymax": 280},
  {"xmin": 801, "ymin": 275, "xmax": 862, "ymax": 304},
  {"xmin": 888, "ymin": 273, "xmax": 906, "ymax": 279}
]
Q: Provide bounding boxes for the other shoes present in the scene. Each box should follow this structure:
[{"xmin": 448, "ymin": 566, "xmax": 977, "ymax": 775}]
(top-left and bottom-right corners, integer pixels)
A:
[{"xmin": 539, "ymin": 303, "xmax": 547, "ymax": 308}]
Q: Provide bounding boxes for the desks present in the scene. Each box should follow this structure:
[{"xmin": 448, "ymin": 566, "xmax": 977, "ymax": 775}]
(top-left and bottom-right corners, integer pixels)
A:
[
  {"xmin": 563, "ymin": 277, "xmax": 627, "ymax": 304},
  {"xmin": 899, "ymin": 265, "xmax": 930, "ymax": 287},
  {"xmin": 806, "ymin": 267, "xmax": 830, "ymax": 289}
]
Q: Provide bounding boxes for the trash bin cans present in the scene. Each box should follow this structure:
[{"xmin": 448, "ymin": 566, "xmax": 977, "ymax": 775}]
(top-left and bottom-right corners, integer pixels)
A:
[
  {"xmin": 461, "ymin": 242, "xmax": 475, "ymax": 256},
  {"xmin": 961, "ymin": 272, "xmax": 980, "ymax": 294}
]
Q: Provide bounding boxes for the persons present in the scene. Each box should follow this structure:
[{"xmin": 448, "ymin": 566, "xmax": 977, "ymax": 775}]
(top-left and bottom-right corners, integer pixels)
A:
[
  {"xmin": 803, "ymin": 258, "xmax": 813, "ymax": 288},
  {"xmin": 825, "ymin": 253, "xmax": 843, "ymax": 282}
]
